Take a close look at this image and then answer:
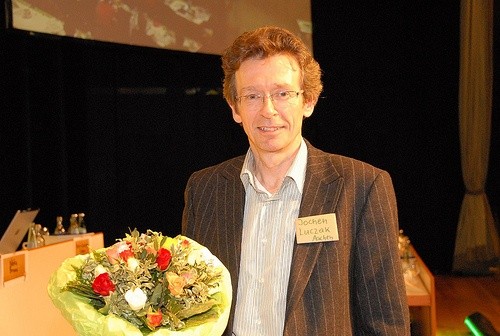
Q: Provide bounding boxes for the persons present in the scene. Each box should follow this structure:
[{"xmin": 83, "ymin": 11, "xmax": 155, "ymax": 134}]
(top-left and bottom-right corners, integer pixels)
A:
[{"xmin": 182, "ymin": 26, "xmax": 410, "ymax": 336}]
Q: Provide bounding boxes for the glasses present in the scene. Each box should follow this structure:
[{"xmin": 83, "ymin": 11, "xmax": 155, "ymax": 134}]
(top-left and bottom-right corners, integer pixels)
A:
[{"xmin": 235, "ymin": 90, "xmax": 304, "ymax": 110}]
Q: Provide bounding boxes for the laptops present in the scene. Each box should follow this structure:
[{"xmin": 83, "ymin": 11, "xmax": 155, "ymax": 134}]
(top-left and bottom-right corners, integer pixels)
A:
[{"xmin": 0, "ymin": 208, "xmax": 41, "ymax": 255}]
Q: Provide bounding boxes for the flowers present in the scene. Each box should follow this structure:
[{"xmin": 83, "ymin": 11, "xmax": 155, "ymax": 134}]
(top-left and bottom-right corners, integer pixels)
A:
[{"xmin": 47, "ymin": 228, "xmax": 233, "ymax": 336}]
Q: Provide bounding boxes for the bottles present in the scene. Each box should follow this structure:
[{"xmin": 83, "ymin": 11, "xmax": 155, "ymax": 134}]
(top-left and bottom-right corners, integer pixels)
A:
[
  {"xmin": 79, "ymin": 212, "xmax": 87, "ymax": 234},
  {"xmin": 36, "ymin": 224, "xmax": 45, "ymax": 247},
  {"xmin": 66, "ymin": 213, "xmax": 79, "ymax": 234},
  {"xmin": 27, "ymin": 222, "xmax": 37, "ymax": 248},
  {"xmin": 398, "ymin": 229, "xmax": 406, "ymax": 259},
  {"xmin": 54, "ymin": 216, "xmax": 66, "ymax": 235}
]
